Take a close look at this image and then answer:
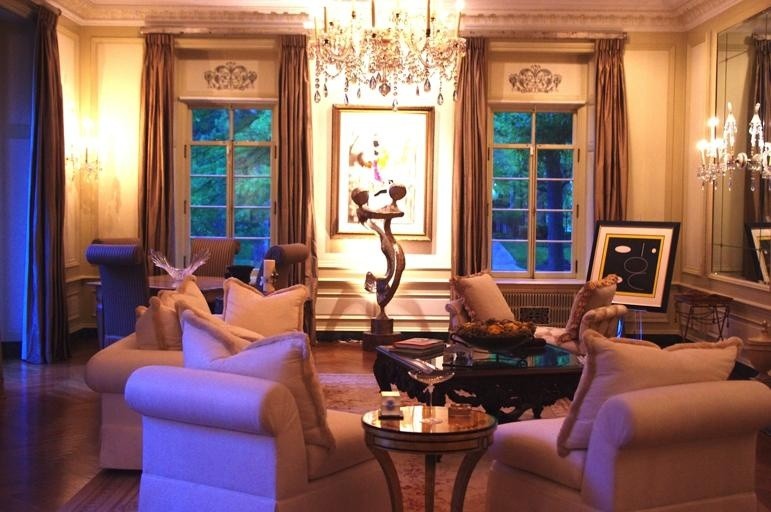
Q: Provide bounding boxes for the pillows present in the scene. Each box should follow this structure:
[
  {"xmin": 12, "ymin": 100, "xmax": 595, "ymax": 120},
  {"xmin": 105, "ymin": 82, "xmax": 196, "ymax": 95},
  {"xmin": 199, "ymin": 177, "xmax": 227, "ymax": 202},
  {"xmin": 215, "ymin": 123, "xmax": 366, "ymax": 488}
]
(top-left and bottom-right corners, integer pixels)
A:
[
  {"xmin": 155, "ymin": 277, "xmax": 218, "ymax": 324},
  {"xmin": 557, "ymin": 329, "xmax": 745, "ymax": 459},
  {"xmin": 135, "ymin": 298, "xmax": 190, "ymax": 352},
  {"xmin": 179, "ymin": 311, "xmax": 339, "ymax": 454},
  {"xmin": 561, "ymin": 274, "xmax": 621, "ymax": 334},
  {"xmin": 176, "ymin": 301, "xmax": 262, "ymax": 341},
  {"xmin": 451, "ymin": 270, "xmax": 517, "ymax": 323},
  {"xmin": 221, "ymin": 274, "xmax": 310, "ymax": 337}
]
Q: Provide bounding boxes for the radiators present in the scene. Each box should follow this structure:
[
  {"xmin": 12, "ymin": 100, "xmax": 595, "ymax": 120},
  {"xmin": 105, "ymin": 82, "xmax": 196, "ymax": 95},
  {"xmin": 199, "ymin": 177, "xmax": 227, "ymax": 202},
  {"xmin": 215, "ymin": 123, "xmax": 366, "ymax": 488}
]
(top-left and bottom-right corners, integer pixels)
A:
[{"xmin": 498, "ymin": 288, "xmax": 580, "ymax": 330}]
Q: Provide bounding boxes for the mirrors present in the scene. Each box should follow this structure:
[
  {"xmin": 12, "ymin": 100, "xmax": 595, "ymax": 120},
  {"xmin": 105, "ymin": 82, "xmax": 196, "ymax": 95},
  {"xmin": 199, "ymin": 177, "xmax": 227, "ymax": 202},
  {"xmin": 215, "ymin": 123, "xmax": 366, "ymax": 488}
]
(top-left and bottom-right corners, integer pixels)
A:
[{"xmin": 702, "ymin": 7, "xmax": 771, "ymax": 293}]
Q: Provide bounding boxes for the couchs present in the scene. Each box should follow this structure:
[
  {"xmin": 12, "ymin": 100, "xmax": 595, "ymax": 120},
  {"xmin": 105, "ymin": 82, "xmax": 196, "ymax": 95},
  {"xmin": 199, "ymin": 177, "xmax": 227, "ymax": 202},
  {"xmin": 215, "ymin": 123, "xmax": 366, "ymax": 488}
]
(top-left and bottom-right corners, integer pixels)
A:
[
  {"xmin": 84, "ymin": 274, "xmax": 313, "ymax": 472},
  {"xmin": 485, "ymin": 378, "xmax": 770, "ymax": 512},
  {"xmin": 121, "ymin": 364, "xmax": 399, "ymax": 511},
  {"xmin": 446, "ymin": 268, "xmax": 630, "ymax": 362}
]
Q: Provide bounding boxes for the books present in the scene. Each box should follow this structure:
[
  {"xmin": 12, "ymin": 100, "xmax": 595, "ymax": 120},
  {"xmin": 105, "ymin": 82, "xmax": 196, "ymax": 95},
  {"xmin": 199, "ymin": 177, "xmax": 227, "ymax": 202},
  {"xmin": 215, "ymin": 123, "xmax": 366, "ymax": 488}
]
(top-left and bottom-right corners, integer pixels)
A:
[{"xmin": 389, "ymin": 338, "xmax": 443, "ymax": 358}]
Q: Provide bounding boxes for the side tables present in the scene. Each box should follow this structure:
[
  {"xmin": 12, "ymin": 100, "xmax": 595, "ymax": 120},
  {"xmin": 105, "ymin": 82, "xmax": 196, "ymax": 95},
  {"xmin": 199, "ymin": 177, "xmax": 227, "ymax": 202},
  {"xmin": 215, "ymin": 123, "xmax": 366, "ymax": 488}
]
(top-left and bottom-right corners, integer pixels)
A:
[{"xmin": 359, "ymin": 404, "xmax": 497, "ymax": 511}]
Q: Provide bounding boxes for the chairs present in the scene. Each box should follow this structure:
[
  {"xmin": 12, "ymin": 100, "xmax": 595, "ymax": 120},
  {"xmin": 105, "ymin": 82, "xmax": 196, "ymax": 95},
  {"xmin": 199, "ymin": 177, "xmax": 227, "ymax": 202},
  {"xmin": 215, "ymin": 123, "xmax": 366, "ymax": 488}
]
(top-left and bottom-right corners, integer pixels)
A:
[
  {"xmin": 213, "ymin": 241, "xmax": 310, "ymax": 317},
  {"xmin": 91, "ymin": 235, "xmax": 144, "ymax": 248},
  {"xmin": 190, "ymin": 235, "xmax": 241, "ymax": 280},
  {"xmin": 86, "ymin": 242, "xmax": 149, "ymax": 353}
]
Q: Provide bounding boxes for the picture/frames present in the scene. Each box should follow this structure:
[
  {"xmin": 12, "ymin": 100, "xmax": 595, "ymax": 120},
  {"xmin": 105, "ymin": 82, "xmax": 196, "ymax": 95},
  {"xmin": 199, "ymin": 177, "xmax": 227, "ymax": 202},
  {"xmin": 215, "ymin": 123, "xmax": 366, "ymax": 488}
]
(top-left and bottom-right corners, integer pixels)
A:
[
  {"xmin": 589, "ymin": 220, "xmax": 682, "ymax": 313},
  {"xmin": 745, "ymin": 221, "xmax": 771, "ymax": 285},
  {"xmin": 331, "ymin": 104, "xmax": 435, "ymax": 243}
]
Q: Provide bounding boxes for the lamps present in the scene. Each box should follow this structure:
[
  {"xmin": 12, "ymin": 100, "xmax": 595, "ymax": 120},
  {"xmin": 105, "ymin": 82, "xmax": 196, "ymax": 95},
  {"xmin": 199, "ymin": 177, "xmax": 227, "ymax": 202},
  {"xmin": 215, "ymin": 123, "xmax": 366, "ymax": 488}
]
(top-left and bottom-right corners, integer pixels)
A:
[
  {"xmin": 303, "ymin": 1, "xmax": 469, "ymax": 112},
  {"xmin": 691, "ymin": 113, "xmax": 748, "ymax": 190},
  {"xmin": 746, "ymin": 102, "xmax": 771, "ymax": 194}
]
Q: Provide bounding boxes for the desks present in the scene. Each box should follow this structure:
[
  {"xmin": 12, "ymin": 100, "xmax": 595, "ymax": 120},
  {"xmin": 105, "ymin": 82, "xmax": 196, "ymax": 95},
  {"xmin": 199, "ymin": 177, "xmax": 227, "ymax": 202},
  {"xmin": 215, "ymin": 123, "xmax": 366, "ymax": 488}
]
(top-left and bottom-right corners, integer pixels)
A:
[{"xmin": 671, "ymin": 291, "xmax": 734, "ymax": 345}]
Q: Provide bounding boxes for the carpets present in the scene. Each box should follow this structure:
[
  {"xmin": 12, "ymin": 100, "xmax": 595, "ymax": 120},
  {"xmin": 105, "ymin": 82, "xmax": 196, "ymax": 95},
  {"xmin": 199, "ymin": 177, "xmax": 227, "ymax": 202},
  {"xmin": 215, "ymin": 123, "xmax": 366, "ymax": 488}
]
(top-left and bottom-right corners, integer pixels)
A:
[{"xmin": 59, "ymin": 366, "xmax": 576, "ymax": 512}]
